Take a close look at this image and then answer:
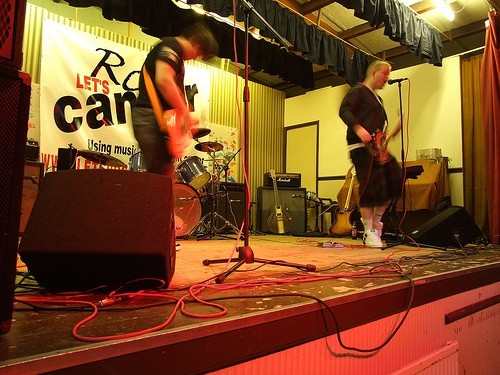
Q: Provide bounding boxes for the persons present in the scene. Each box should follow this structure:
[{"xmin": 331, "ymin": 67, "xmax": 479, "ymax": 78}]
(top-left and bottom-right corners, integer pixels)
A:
[
  {"xmin": 339, "ymin": 61, "xmax": 403, "ymax": 247},
  {"xmin": 132, "ymin": 23, "xmax": 218, "ymax": 177}
]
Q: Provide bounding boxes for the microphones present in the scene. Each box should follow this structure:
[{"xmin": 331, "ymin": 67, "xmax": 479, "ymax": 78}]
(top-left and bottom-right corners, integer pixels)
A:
[
  {"xmin": 291, "ymin": 194, "xmax": 301, "ymax": 198},
  {"xmin": 388, "ymin": 78, "xmax": 408, "ymax": 85}
]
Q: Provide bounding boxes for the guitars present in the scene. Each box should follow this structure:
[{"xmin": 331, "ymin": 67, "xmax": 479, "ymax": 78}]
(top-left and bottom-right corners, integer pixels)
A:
[
  {"xmin": 267, "ymin": 169, "xmax": 293, "ymax": 234},
  {"xmin": 370, "ymin": 115, "xmax": 401, "ymax": 164},
  {"xmin": 161, "ymin": 108, "xmax": 207, "ymax": 159},
  {"xmin": 329, "ymin": 166, "xmax": 357, "ymax": 236}
]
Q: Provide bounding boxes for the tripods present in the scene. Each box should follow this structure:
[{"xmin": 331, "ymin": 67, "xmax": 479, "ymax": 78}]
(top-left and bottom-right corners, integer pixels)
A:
[
  {"xmin": 379, "ymin": 82, "xmax": 446, "ymax": 250},
  {"xmin": 183, "ymin": 148, "xmax": 246, "ymax": 238},
  {"xmin": 202, "ymin": 0, "xmax": 317, "ymax": 282}
]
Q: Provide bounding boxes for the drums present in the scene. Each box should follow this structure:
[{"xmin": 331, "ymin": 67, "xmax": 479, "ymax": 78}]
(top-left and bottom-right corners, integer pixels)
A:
[
  {"xmin": 172, "ymin": 183, "xmax": 203, "ymax": 239},
  {"xmin": 176, "ymin": 156, "xmax": 211, "ymax": 190},
  {"xmin": 129, "ymin": 151, "xmax": 141, "ymax": 170}
]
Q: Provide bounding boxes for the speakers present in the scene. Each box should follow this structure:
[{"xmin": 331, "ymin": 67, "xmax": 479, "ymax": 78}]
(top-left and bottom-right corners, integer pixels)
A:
[
  {"xmin": 408, "ymin": 205, "xmax": 487, "ymax": 249},
  {"xmin": 256, "ymin": 187, "xmax": 308, "ymax": 236},
  {"xmin": 203, "ymin": 182, "xmax": 247, "ymax": 236},
  {"xmin": 19, "ymin": 168, "xmax": 176, "ymax": 287}
]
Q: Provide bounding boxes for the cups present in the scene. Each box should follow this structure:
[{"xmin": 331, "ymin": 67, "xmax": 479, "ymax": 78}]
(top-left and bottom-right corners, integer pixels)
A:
[{"xmin": 415, "ymin": 148, "xmax": 442, "ymax": 160}]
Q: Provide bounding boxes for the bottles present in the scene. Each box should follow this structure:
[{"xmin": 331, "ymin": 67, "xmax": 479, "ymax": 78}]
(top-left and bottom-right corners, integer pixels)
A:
[{"xmin": 352, "ymin": 221, "xmax": 357, "ymax": 239}]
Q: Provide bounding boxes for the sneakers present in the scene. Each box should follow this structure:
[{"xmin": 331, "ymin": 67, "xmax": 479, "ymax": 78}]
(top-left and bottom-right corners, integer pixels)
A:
[
  {"xmin": 363, "ymin": 229, "xmax": 383, "ymax": 247},
  {"xmin": 370, "ymin": 214, "xmax": 384, "ymax": 237}
]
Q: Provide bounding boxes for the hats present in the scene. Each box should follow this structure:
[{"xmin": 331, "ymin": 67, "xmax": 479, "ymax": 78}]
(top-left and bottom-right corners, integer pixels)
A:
[{"xmin": 188, "ymin": 20, "xmax": 219, "ymax": 61}]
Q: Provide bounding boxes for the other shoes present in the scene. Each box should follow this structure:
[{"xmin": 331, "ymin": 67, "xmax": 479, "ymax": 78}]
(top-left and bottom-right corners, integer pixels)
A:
[{"xmin": 175, "ymin": 243, "xmax": 181, "ymax": 251}]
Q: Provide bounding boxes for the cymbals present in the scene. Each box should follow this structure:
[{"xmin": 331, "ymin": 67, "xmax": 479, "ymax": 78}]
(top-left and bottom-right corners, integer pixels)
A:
[
  {"xmin": 191, "ymin": 128, "xmax": 211, "ymax": 138},
  {"xmin": 77, "ymin": 150, "xmax": 127, "ymax": 167},
  {"xmin": 204, "ymin": 158, "xmax": 223, "ymax": 161},
  {"xmin": 195, "ymin": 142, "xmax": 223, "ymax": 152}
]
